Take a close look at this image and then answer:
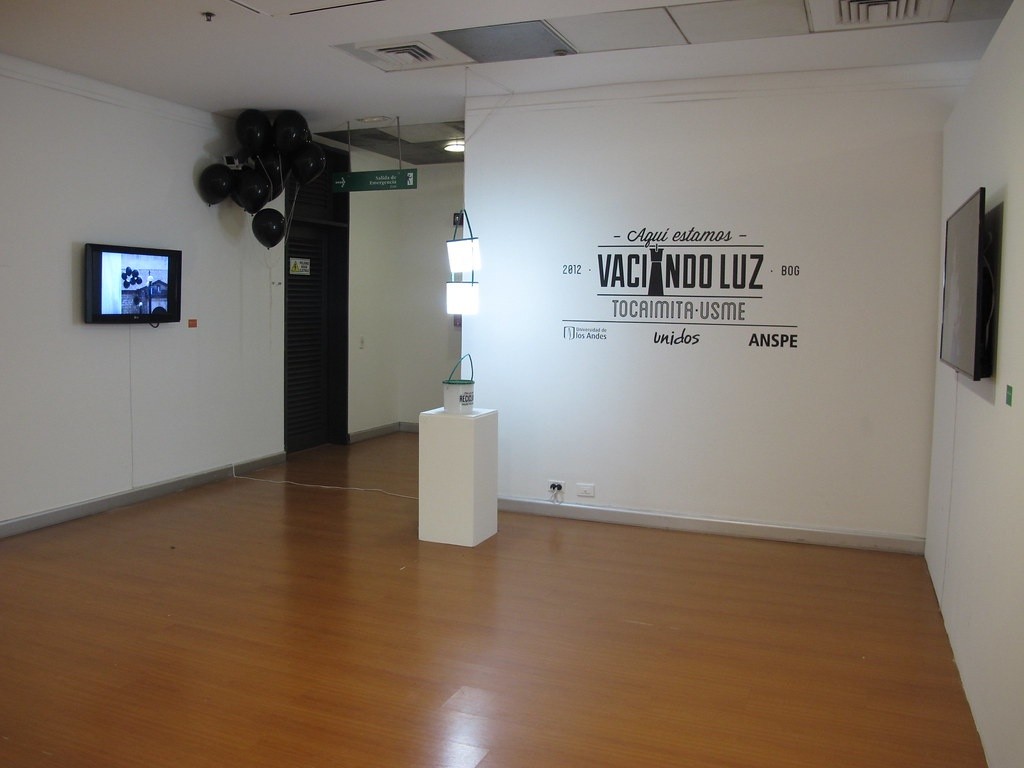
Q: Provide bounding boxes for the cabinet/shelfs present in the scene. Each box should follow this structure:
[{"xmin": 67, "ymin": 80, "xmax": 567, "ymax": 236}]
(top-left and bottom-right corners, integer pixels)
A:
[{"xmin": 419, "ymin": 408, "xmax": 499, "ymax": 548}]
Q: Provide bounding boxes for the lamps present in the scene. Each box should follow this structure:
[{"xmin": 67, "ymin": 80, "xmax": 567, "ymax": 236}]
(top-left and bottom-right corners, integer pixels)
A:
[
  {"xmin": 442, "ymin": 133, "xmax": 464, "ymax": 153},
  {"xmin": 445, "ymin": 207, "xmax": 481, "ymax": 315}
]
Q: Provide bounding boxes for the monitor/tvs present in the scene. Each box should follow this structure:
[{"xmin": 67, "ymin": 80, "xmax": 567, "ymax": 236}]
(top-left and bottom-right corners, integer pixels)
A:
[
  {"xmin": 938, "ymin": 186, "xmax": 989, "ymax": 381},
  {"xmin": 85, "ymin": 242, "xmax": 182, "ymax": 323}
]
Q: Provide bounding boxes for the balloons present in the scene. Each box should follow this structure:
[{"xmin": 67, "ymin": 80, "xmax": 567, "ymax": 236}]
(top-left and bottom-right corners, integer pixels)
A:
[
  {"xmin": 201, "ymin": 162, "xmax": 230, "ymax": 207},
  {"xmin": 251, "ymin": 206, "xmax": 286, "ymax": 248},
  {"xmin": 230, "ymin": 108, "xmax": 327, "ymax": 212},
  {"xmin": 121, "ymin": 267, "xmax": 142, "ymax": 288},
  {"xmin": 134, "ymin": 296, "xmax": 139, "ymax": 305}
]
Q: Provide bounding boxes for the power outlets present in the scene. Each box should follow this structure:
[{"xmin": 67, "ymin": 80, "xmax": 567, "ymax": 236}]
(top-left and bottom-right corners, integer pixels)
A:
[{"xmin": 548, "ymin": 480, "xmax": 566, "ymax": 494}]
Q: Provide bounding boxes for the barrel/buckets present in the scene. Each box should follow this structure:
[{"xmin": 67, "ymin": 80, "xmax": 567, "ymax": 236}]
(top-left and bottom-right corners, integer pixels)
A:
[{"xmin": 442, "ymin": 353, "xmax": 475, "ymax": 414}]
